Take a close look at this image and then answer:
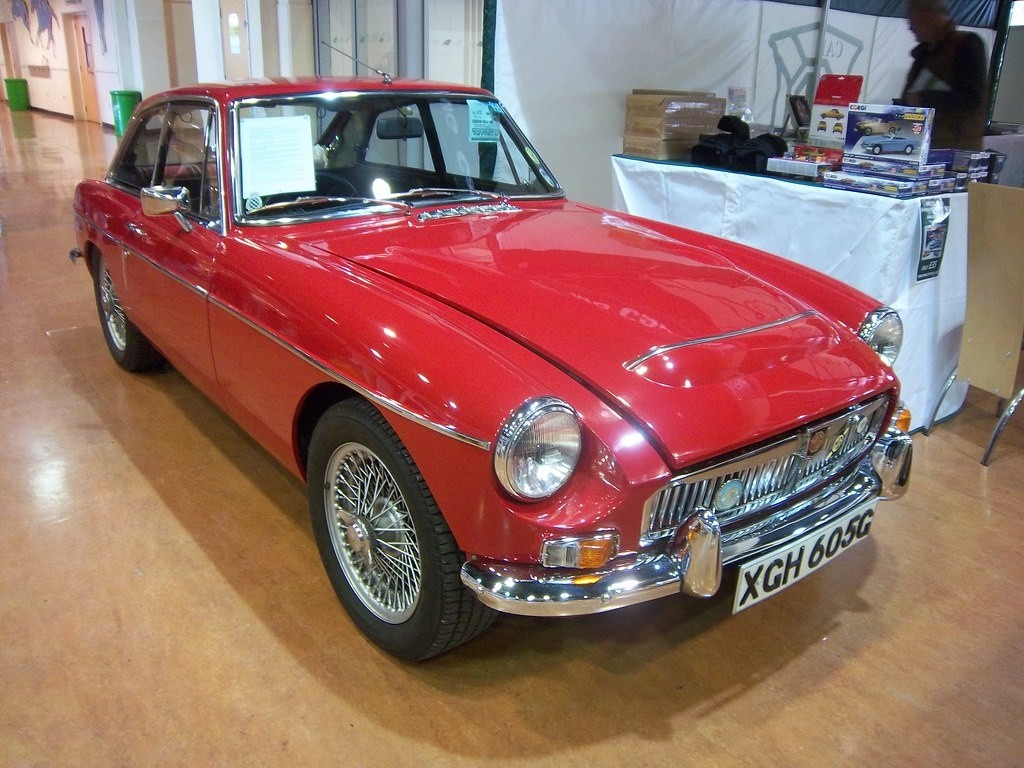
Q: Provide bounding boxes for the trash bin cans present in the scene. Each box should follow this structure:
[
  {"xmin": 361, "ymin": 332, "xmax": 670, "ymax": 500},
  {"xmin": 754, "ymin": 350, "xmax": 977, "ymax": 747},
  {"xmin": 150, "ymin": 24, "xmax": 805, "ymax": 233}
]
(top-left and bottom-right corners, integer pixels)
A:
[
  {"xmin": 4, "ymin": 79, "xmax": 28, "ymax": 111},
  {"xmin": 109, "ymin": 91, "xmax": 143, "ymax": 136}
]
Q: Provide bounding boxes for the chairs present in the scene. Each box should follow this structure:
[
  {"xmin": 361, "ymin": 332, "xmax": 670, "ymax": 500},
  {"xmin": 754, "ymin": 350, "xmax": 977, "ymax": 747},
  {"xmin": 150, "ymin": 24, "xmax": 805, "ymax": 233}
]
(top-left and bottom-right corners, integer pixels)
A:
[{"xmin": 922, "ymin": 182, "xmax": 1024, "ymax": 467}]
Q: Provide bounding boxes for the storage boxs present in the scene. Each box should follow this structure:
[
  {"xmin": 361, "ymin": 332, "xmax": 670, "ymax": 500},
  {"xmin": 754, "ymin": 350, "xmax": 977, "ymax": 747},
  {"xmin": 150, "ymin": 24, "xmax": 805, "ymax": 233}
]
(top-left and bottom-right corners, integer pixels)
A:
[
  {"xmin": 620, "ymin": 89, "xmax": 726, "ymax": 161},
  {"xmin": 767, "ymin": 73, "xmax": 1008, "ymax": 200}
]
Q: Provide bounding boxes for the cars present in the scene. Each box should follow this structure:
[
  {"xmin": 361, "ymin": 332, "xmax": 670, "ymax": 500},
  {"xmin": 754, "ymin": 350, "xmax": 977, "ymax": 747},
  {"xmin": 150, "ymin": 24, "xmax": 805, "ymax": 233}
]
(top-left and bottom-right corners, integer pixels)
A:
[
  {"xmin": 831, "ymin": 123, "xmax": 844, "ymax": 135},
  {"xmin": 816, "ymin": 121, "xmax": 828, "ymax": 132},
  {"xmin": 819, "ymin": 108, "xmax": 845, "ymax": 121}
]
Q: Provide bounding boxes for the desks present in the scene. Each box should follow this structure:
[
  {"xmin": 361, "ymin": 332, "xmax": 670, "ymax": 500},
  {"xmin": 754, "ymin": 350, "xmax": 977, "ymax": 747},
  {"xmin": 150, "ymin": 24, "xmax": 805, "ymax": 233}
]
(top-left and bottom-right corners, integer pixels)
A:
[{"xmin": 611, "ymin": 152, "xmax": 969, "ymax": 434}]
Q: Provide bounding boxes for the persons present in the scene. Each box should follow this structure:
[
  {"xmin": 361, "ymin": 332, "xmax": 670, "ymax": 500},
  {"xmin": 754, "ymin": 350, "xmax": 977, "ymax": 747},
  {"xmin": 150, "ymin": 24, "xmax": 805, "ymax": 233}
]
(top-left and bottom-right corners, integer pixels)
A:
[{"xmin": 898, "ymin": 0, "xmax": 988, "ymax": 152}]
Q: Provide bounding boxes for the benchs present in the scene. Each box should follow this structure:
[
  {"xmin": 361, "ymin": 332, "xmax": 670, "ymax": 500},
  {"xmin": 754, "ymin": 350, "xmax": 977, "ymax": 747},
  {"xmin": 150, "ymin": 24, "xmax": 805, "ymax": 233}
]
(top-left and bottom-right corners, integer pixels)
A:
[{"xmin": 133, "ymin": 161, "xmax": 217, "ymax": 214}]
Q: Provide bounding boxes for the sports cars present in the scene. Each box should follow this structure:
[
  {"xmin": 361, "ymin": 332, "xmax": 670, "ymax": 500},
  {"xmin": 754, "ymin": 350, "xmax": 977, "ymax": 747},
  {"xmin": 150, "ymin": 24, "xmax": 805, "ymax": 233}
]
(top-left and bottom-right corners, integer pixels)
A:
[
  {"xmin": 65, "ymin": 73, "xmax": 915, "ymax": 667},
  {"xmin": 855, "ymin": 115, "xmax": 902, "ymax": 136},
  {"xmin": 860, "ymin": 131, "xmax": 921, "ymax": 155}
]
